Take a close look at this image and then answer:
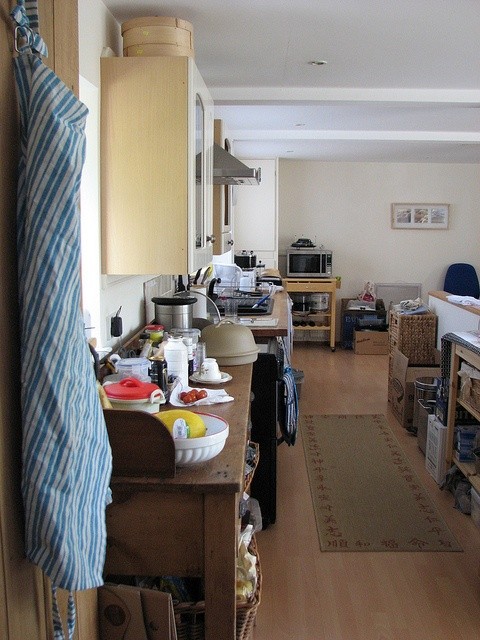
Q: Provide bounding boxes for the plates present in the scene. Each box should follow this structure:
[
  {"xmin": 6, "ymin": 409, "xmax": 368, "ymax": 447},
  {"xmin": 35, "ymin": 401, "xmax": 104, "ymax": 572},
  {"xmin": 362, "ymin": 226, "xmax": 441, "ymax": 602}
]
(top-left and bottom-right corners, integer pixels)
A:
[{"xmin": 189, "ymin": 371, "xmax": 233, "ymax": 384}]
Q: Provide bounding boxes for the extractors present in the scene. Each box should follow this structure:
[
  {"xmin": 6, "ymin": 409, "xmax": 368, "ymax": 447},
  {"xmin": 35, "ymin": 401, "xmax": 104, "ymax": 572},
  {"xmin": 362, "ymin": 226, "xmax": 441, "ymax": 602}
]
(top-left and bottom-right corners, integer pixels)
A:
[{"xmin": 213, "ymin": 143, "xmax": 262, "ymax": 187}]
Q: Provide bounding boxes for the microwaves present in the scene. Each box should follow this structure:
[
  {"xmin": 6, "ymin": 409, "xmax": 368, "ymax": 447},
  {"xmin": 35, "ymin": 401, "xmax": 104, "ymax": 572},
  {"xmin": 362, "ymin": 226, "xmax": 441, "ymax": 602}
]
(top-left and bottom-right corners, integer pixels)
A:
[{"xmin": 286, "ymin": 248, "xmax": 332, "ymax": 278}]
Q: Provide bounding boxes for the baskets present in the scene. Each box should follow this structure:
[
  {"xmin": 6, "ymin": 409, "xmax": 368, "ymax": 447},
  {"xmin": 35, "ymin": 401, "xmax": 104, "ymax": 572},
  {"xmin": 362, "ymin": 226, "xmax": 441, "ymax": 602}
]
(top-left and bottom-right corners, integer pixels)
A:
[
  {"xmin": 136, "ymin": 523, "xmax": 262, "ymax": 640},
  {"xmin": 242, "ymin": 439, "xmax": 260, "ymax": 496},
  {"xmin": 460, "ymin": 361, "xmax": 480, "ymax": 413},
  {"xmin": 389, "ymin": 309, "xmax": 436, "ymax": 366}
]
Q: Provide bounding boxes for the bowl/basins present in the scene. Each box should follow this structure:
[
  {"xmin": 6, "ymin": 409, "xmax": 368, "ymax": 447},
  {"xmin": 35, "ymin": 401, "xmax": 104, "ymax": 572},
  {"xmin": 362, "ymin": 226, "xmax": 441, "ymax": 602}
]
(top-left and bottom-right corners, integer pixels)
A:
[
  {"xmin": 151, "ymin": 410, "xmax": 230, "ymax": 467},
  {"xmin": 199, "ymin": 358, "xmax": 221, "ymax": 381}
]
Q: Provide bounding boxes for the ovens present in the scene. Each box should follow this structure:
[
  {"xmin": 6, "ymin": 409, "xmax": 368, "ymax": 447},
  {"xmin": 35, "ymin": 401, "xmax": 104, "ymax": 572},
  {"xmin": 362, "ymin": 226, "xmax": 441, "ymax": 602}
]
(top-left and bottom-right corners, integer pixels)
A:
[{"xmin": 243, "ymin": 309, "xmax": 281, "ymax": 335}]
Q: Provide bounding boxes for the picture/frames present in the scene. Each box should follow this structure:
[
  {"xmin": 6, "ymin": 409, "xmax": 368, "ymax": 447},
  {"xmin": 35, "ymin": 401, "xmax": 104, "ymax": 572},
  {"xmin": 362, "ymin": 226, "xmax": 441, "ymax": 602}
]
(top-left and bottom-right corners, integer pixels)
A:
[{"xmin": 390, "ymin": 202, "xmax": 451, "ymax": 232}]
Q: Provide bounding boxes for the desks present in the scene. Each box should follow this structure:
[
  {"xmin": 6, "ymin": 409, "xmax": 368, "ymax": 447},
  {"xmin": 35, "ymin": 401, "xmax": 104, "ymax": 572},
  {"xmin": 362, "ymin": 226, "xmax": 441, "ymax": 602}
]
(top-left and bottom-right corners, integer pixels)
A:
[
  {"xmin": 282, "ymin": 278, "xmax": 338, "ymax": 351},
  {"xmin": 99, "ymin": 362, "xmax": 253, "ymax": 638}
]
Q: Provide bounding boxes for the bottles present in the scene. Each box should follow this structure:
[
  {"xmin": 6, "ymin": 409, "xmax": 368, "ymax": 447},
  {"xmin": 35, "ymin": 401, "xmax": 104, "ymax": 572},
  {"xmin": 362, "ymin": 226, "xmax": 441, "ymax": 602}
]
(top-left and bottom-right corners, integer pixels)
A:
[{"xmin": 163, "ymin": 335, "xmax": 189, "ymax": 388}]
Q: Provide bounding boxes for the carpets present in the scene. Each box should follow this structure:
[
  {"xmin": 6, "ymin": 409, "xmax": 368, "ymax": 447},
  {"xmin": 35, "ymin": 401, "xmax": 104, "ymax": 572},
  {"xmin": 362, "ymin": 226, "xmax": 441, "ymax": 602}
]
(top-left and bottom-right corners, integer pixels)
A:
[{"xmin": 298, "ymin": 411, "xmax": 465, "ymax": 554}]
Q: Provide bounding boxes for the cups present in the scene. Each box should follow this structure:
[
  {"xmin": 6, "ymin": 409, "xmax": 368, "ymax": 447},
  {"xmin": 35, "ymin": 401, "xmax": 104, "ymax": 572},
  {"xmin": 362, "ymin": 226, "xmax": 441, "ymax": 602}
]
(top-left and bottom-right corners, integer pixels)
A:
[
  {"xmin": 195, "ymin": 342, "xmax": 206, "ymax": 372},
  {"xmin": 225, "ymin": 299, "xmax": 238, "ymax": 320}
]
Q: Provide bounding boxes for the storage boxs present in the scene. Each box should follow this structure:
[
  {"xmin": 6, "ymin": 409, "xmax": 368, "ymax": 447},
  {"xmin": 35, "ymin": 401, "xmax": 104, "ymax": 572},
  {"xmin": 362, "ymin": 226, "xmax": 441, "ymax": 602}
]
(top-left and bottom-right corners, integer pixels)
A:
[
  {"xmin": 353, "ymin": 329, "xmax": 388, "ymax": 356},
  {"xmin": 424, "ymin": 414, "xmax": 449, "ymax": 490},
  {"xmin": 389, "ymin": 347, "xmax": 441, "ymax": 428}
]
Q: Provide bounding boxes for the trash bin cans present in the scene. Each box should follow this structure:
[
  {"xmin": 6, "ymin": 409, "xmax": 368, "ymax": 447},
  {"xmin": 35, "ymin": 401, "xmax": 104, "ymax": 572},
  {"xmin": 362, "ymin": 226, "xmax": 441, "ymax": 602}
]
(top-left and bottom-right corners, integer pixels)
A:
[
  {"xmin": 413, "ymin": 376, "xmax": 441, "ymax": 437},
  {"xmin": 293, "ymin": 370, "xmax": 304, "ymax": 400}
]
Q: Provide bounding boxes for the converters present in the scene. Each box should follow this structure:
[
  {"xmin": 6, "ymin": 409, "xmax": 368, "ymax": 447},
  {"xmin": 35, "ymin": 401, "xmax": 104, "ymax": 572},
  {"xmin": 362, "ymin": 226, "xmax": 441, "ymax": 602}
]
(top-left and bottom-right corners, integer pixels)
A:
[{"xmin": 112, "ymin": 316, "xmax": 122, "ymax": 337}]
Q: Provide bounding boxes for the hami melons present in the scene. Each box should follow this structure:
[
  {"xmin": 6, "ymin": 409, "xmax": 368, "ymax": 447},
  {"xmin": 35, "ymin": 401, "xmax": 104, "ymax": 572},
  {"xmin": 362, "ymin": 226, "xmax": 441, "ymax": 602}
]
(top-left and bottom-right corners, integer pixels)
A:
[{"xmin": 155, "ymin": 409, "xmax": 208, "ymax": 438}]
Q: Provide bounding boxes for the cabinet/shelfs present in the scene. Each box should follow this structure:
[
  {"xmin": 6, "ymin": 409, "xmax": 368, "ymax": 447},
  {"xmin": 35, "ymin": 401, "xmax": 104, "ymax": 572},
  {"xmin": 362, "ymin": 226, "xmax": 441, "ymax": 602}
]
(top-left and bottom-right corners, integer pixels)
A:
[
  {"xmin": 437, "ymin": 328, "xmax": 480, "ymax": 507},
  {"xmin": 211, "ymin": 118, "xmax": 235, "ymax": 257},
  {"xmin": 97, "ymin": 53, "xmax": 217, "ymax": 276}
]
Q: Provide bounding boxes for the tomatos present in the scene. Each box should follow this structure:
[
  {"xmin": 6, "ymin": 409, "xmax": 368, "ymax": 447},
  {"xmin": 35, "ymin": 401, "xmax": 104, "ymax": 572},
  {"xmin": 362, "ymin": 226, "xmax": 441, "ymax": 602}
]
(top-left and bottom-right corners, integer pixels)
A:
[{"xmin": 180, "ymin": 389, "xmax": 207, "ymax": 404}]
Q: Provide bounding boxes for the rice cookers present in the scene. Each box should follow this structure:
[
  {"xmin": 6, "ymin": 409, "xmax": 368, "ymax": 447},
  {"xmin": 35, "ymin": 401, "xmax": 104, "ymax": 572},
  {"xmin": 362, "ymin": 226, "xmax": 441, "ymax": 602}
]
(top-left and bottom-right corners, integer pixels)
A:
[{"xmin": 308, "ymin": 292, "xmax": 330, "ymax": 312}]
